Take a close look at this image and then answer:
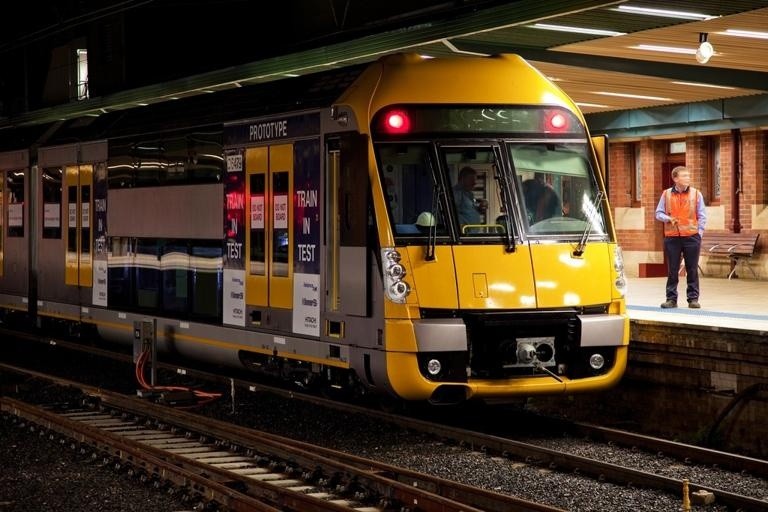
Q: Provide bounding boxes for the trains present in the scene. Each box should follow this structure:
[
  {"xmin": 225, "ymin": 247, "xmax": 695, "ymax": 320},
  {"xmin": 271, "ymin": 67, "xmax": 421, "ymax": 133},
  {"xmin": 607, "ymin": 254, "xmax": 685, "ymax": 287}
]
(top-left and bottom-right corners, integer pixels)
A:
[{"xmin": 0, "ymin": 48, "xmax": 630, "ymax": 407}]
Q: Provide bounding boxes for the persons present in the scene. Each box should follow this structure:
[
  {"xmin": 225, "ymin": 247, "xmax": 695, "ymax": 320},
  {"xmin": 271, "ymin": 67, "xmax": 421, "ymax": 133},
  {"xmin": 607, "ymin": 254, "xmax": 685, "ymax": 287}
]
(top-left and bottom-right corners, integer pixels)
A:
[
  {"xmin": 450, "ymin": 166, "xmax": 488, "ymax": 238},
  {"xmin": 523, "ymin": 180, "xmax": 562, "ymax": 226},
  {"xmin": 654, "ymin": 165, "xmax": 706, "ymax": 309}
]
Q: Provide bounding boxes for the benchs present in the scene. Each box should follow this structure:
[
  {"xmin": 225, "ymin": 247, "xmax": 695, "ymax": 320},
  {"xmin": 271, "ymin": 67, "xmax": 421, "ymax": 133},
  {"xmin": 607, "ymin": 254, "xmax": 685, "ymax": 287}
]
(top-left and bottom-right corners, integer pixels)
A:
[{"xmin": 676, "ymin": 227, "xmax": 761, "ymax": 279}]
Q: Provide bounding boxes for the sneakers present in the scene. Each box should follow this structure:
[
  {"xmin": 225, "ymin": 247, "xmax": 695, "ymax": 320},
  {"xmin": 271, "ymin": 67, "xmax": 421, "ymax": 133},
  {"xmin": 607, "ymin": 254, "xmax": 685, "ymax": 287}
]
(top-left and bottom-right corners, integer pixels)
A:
[
  {"xmin": 687, "ymin": 298, "xmax": 702, "ymax": 309},
  {"xmin": 660, "ymin": 298, "xmax": 679, "ymax": 309}
]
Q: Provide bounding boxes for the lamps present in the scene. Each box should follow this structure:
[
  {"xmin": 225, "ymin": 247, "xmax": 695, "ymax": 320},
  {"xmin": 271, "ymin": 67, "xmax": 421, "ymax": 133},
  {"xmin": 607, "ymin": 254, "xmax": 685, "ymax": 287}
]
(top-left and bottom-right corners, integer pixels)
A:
[{"xmin": 693, "ymin": 28, "xmax": 713, "ymax": 65}]
